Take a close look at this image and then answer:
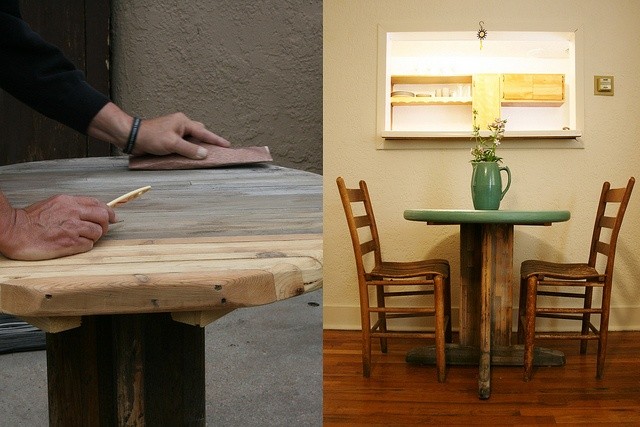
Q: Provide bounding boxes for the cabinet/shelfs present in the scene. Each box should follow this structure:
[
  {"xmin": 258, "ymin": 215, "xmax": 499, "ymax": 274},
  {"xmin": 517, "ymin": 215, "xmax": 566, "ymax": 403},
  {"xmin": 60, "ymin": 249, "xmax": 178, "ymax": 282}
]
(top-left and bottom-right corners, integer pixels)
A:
[{"xmin": 391, "ymin": 73, "xmax": 567, "ymax": 107}]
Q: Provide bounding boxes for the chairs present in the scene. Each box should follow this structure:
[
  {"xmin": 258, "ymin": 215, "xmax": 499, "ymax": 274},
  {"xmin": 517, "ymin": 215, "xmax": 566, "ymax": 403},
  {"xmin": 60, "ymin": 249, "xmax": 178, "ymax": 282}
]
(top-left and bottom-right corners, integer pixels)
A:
[
  {"xmin": 516, "ymin": 175, "xmax": 636, "ymax": 382},
  {"xmin": 336, "ymin": 176, "xmax": 451, "ymax": 384}
]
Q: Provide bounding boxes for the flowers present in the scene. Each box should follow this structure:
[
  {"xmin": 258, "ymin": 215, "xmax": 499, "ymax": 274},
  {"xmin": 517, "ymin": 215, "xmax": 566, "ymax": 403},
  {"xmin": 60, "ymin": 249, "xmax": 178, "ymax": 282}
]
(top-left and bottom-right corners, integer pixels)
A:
[{"xmin": 468, "ymin": 105, "xmax": 507, "ymax": 165}]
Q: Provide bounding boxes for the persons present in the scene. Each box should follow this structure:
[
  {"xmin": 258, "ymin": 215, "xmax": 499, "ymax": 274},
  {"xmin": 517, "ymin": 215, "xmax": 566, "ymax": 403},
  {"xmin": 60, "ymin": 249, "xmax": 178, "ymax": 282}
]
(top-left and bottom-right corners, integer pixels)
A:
[{"xmin": 1, "ymin": 14, "xmax": 231, "ymax": 261}]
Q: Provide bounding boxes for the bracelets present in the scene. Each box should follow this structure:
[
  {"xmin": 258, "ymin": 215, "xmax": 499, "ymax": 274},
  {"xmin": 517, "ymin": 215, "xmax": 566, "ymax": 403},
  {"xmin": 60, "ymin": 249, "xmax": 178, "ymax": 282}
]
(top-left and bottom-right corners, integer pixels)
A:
[{"xmin": 121, "ymin": 117, "xmax": 141, "ymax": 153}]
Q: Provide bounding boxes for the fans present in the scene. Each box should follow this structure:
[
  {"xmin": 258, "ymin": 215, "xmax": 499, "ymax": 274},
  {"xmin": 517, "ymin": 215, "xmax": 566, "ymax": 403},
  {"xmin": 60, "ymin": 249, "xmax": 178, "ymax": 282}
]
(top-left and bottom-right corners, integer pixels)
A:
[{"xmin": 1, "ymin": 154, "xmax": 323, "ymax": 426}]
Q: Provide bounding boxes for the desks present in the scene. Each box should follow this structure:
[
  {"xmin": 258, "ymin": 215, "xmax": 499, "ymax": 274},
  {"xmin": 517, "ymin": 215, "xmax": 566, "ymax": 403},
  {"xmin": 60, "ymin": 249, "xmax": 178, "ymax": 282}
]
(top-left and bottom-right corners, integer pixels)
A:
[{"xmin": 403, "ymin": 208, "xmax": 571, "ymax": 401}]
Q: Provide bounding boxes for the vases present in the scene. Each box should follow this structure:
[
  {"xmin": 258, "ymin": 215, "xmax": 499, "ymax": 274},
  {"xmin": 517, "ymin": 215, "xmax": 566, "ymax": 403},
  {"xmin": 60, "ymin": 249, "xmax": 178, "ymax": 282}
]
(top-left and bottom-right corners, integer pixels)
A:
[{"xmin": 470, "ymin": 159, "xmax": 511, "ymax": 211}]
